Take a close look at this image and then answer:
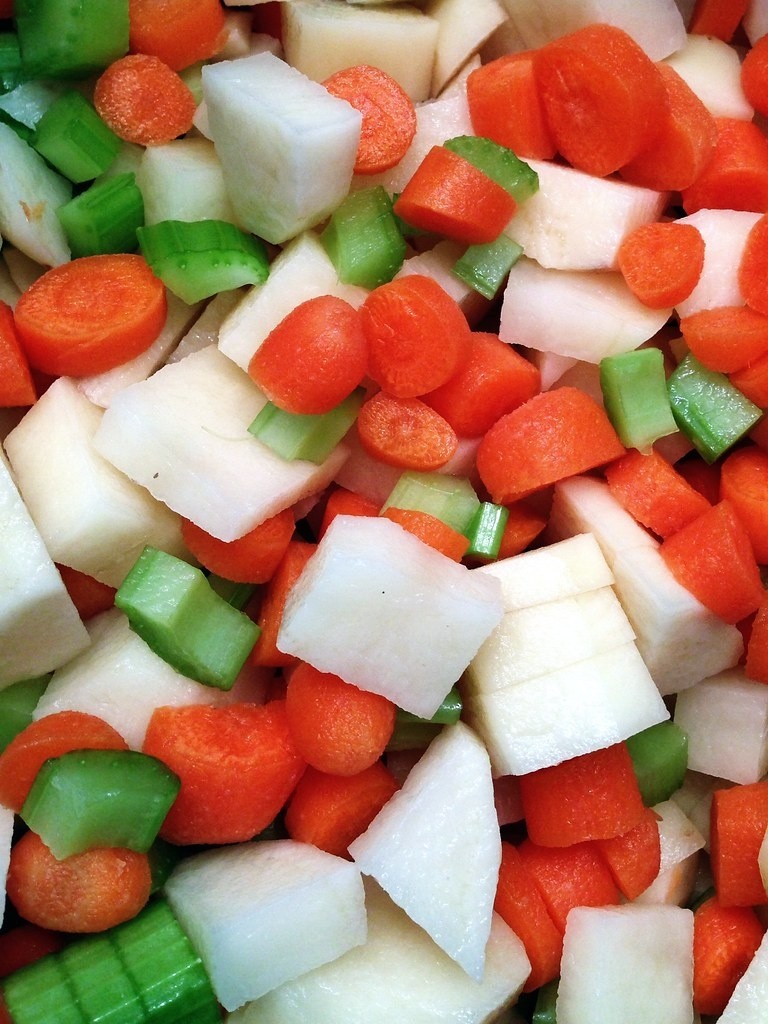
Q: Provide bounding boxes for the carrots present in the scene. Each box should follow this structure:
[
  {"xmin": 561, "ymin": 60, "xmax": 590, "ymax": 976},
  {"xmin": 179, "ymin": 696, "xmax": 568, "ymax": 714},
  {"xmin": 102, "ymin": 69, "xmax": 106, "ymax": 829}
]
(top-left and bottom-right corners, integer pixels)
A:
[{"xmin": 0, "ymin": 0, "xmax": 768, "ymax": 1010}]
